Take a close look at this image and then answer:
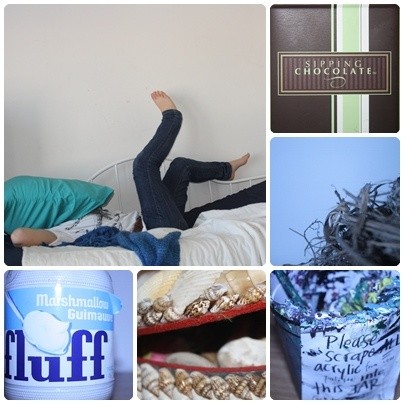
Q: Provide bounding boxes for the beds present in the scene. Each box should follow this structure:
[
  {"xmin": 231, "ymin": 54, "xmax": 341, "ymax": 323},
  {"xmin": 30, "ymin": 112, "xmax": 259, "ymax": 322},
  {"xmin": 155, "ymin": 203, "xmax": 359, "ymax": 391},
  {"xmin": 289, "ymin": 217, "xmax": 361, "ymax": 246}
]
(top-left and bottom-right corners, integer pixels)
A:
[{"xmin": 4, "ymin": 156, "xmax": 266, "ymax": 266}]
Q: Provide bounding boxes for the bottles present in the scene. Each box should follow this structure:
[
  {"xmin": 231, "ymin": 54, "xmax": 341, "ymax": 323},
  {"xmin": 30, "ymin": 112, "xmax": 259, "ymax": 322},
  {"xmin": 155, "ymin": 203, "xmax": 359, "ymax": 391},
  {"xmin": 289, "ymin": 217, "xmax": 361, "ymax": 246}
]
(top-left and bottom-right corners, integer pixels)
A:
[{"xmin": 5, "ymin": 268, "xmax": 114, "ymax": 397}]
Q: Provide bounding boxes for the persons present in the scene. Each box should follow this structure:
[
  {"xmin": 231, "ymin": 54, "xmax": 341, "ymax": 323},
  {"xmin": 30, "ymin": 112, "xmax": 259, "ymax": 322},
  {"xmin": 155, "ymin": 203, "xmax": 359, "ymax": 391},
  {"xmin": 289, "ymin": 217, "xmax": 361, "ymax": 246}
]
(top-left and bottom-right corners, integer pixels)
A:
[{"xmin": 10, "ymin": 90, "xmax": 250, "ymax": 248}]
